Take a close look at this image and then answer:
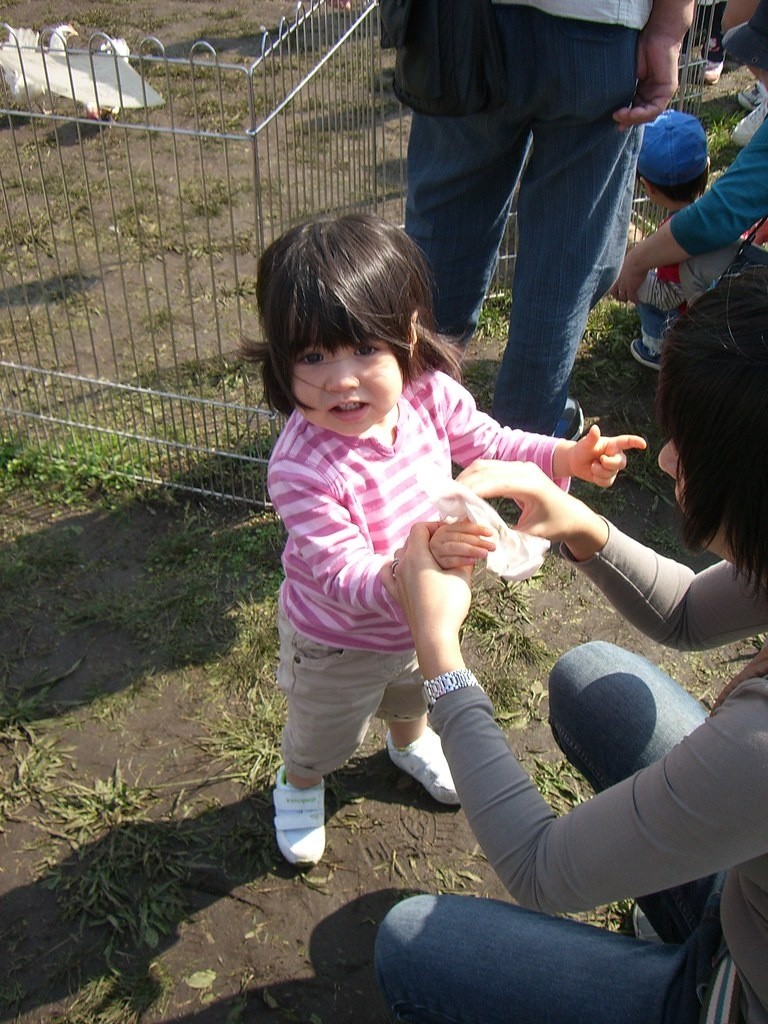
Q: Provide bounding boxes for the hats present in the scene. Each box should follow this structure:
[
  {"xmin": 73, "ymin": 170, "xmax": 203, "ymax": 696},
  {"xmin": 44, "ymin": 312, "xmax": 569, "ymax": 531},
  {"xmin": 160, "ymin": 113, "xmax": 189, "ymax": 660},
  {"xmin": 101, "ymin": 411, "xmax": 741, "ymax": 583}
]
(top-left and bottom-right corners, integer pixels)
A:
[
  {"xmin": 722, "ymin": 0, "xmax": 768, "ymax": 70},
  {"xmin": 636, "ymin": 110, "xmax": 709, "ymax": 186}
]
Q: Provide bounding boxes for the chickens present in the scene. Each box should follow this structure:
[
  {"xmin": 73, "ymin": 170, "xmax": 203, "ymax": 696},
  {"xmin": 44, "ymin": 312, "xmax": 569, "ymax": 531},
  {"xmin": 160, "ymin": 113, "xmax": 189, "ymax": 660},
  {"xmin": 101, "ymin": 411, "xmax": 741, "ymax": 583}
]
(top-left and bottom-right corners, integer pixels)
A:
[{"xmin": 1, "ymin": 24, "xmax": 166, "ymax": 119}]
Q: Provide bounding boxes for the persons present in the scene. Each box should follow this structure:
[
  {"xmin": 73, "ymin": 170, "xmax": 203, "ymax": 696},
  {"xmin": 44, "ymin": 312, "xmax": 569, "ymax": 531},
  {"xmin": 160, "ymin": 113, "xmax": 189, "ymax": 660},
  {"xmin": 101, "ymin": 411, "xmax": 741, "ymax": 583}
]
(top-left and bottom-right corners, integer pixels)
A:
[
  {"xmin": 236, "ymin": 212, "xmax": 649, "ymax": 870},
  {"xmin": 403, "ymin": 0, "xmax": 695, "ymax": 495},
  {"xmin": 608, "ymin": 0, "xmax": 768, "ymax": 371},
  {"xmin": 308, "ymin": 265, "xmax": 768, "ymax": 1024}
]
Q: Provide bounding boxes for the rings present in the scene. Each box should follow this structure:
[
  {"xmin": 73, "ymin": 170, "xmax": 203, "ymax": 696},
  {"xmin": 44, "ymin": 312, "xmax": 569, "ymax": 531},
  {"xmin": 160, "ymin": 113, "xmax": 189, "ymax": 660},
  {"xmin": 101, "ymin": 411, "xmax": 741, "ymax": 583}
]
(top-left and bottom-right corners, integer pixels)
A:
[
  {"xmin": 619, "ymin": 290, "xmax": 625, "ymax": 294},
  {"xmin": 391, "ymin": 558, "xmax": 399, "ymax": 578}
]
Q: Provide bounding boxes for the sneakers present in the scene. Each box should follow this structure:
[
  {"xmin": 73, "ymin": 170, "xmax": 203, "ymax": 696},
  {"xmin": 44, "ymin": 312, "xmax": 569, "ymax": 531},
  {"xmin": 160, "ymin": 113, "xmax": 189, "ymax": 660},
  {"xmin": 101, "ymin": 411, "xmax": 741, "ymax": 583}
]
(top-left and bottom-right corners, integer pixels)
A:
[
  {"xmin": 273, "ymin": 767, "xmax": 325, "ymax": 868},
  {"xmin": 631, "ymin": 339, "xmax": 661, "ymax": 370},
  {"xmin": 699, "ymin": 57, "xmax": 724, "ymax": 84},
  {"xmin": 552, "ymin": 397, "xmax": 584, "ymax": 441},
  {"xmin": 386, "ymin": 726, "xmax": 460, "ymax": 803},
  {"xmin": 732, "ymin": 100, "xmax": 768, "ymax": 146},
  {"xmin": 738, "ymin": 82, "xmax": 768, "ymax": 110}
]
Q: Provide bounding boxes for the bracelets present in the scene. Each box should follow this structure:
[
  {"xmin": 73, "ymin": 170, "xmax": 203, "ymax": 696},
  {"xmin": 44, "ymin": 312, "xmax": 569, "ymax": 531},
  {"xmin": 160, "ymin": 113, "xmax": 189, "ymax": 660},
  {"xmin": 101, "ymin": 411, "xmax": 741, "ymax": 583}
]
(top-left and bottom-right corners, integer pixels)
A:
[{"xmin": 420, "ymin": 668, "xmax": 479, "ymax": 712}]
